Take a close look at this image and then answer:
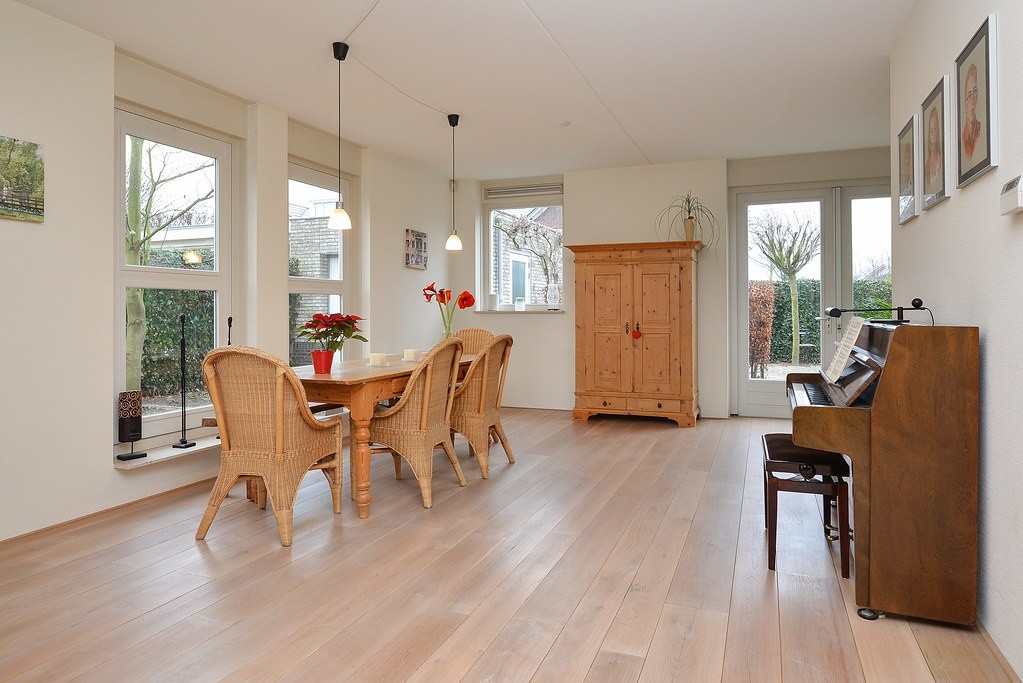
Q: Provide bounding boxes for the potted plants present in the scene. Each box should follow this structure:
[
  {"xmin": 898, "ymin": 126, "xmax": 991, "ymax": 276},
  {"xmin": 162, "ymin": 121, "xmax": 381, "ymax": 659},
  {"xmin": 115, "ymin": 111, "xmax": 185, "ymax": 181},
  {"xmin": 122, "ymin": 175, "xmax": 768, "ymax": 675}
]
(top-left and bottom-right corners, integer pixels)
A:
[{"xmin": 654, "ymin": 191, "xmax": 720, "ymax": 254}]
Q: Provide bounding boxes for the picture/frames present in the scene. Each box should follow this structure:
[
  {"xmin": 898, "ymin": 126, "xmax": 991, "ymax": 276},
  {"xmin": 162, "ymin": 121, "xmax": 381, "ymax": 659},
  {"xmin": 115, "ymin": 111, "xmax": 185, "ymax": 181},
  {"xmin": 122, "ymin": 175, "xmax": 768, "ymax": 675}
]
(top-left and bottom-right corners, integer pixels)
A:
[
  {"xmin": 898, "ymin": 114, "xmax": 920, "ymax": 225},
  {"xmin": 954, "ymin": 13, "xmax": 999, "ymax": 189},
  {"xmin": 921, "ymin": 75, "xmax": 952, "ymax": 211}
]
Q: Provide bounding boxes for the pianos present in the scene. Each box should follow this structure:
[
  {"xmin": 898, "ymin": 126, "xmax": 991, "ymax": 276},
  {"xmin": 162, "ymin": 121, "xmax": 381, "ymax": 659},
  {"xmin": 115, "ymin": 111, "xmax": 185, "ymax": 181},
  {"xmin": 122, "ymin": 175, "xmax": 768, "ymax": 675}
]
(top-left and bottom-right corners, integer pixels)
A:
[{"xmin": 786, "ymin": 318, "xmax": 980, "ymax": 632}]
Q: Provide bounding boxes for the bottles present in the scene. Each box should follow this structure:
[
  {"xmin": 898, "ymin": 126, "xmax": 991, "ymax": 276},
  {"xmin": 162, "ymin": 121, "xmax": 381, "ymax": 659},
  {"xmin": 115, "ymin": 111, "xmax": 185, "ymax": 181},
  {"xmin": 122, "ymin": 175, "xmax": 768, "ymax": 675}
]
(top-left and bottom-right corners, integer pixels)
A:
[{"xmin": 515, "ymin": 297, "xmax": 525, "ymax": 311}]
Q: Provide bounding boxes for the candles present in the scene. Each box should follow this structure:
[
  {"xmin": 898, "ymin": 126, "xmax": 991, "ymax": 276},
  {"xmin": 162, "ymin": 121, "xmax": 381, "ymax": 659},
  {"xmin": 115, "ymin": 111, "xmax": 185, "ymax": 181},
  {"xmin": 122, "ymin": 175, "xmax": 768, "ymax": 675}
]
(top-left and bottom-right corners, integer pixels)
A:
[
  {"xmin": 369, "ymin": 353, "xmax": 386, "ymax": 364},
  {"xmin": 404, "ymin": 349, "xmax": 420, "ymax": 359}
]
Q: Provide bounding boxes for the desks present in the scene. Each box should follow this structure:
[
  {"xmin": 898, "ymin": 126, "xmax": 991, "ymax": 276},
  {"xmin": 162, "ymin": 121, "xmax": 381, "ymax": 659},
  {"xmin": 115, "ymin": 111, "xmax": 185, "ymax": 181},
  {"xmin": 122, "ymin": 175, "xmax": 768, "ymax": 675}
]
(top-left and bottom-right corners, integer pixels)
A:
[{"xmin": 244, "ymin": 352, "xmax": 479, "ymax": 518}]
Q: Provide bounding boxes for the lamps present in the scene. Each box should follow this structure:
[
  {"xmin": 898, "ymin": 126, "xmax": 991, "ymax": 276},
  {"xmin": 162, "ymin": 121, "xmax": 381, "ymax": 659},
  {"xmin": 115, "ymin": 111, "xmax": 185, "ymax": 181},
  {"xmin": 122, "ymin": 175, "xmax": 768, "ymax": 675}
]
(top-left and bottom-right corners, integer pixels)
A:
[
  {"xmin": 484, "ymin": 182, "xmax": 564, "ymax": 199},
  {"xmin": 445, "ymin": 114, "xmax": 462, "ymax": 251},
  {"xmin": 116, "ymin": 390, "xmax": 147, "ymax": 460},
  {"xmin": 327, "ymin": 41, "xmax": 351, "ymax": 230}
]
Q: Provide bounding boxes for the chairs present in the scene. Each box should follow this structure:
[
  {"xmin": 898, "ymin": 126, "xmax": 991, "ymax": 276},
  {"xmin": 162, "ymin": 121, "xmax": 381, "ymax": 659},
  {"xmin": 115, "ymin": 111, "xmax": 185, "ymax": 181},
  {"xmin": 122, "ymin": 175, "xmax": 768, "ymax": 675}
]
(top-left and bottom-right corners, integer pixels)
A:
[{"xmin": 195, "ymin": 329, "xmax": 514, "ymax": 549}]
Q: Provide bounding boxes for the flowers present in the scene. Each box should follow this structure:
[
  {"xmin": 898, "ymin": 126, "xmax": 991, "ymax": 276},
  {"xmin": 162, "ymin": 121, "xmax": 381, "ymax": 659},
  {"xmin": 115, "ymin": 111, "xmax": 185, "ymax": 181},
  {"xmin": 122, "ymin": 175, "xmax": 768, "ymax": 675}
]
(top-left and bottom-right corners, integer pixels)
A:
[
  {"xmin": 422, "ymin": 282, "xmax": 476, "ymax": 340},
  {"xmin": 296, "ymin": 312, "xmax": 368, "ymax": 352}
]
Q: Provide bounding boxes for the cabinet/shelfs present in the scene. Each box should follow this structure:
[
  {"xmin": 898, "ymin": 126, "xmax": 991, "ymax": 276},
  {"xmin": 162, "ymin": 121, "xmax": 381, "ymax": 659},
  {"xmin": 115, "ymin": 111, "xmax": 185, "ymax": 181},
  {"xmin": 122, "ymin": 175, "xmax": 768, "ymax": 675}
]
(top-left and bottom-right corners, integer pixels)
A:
[{"xmin": 564, "ymin": 240, "xmax": 707, "ymax": 427}]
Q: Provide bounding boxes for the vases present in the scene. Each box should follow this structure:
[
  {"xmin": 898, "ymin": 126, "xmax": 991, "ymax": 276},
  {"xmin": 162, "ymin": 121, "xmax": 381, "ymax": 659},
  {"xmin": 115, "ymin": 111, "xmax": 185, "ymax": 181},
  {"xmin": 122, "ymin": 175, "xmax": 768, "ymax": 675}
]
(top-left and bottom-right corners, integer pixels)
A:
[
  {"xmin": 442, "ymin": 333, "xmax": 452, "ymax": 340},
  {"xmin": 310, "ymin": 350, "xmax": 333, "ymax": 374}
]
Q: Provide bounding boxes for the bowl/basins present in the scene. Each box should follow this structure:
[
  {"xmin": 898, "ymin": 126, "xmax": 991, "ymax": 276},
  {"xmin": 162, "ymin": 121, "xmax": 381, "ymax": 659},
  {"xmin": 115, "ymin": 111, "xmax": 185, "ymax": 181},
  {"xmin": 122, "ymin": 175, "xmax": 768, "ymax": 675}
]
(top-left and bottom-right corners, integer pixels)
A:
[
  {"xmin": 403, "ymin": 349, "xmax": 420, "ymax": 360},
  {"xmin": 369, "ymin": 353, "xmax": 387, "ymax": 364}
]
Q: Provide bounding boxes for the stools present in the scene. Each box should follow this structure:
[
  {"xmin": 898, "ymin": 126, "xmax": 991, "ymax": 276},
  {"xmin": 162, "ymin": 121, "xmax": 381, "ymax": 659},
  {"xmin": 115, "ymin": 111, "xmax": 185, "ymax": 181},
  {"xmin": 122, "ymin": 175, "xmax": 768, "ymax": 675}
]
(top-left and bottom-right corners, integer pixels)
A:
[{"xmin": 761, "ymin": 433, "xmax": 850, "ymax": 578}]
